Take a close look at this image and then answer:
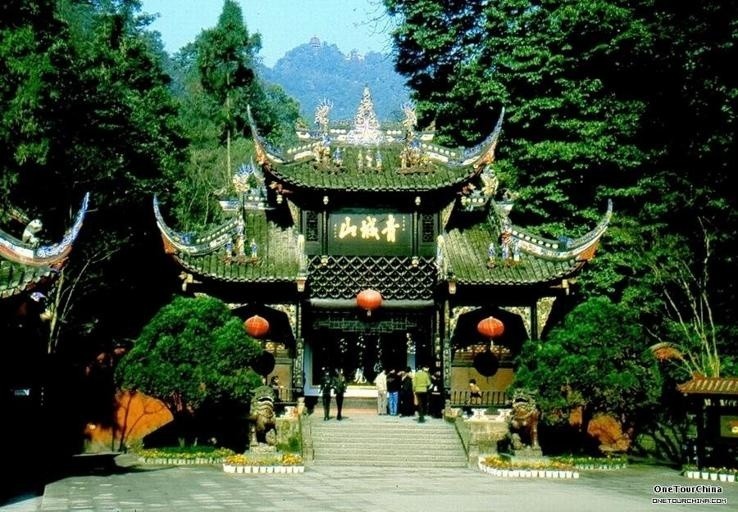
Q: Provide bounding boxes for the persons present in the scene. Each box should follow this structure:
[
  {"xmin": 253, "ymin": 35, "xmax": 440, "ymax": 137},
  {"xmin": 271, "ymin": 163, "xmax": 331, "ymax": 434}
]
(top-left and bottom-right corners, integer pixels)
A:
[
  {"xmin": 333, "ymin": 367, "xmax": 347, "ymax": 420},
  {"xmin": 318, "ymin": 369, "xmax": 334, "ymax": 421},
  {"xmin": 464, "ymin": 378, "xmax": 483, "ymax": 404},
  {"xmin": 268, "ymin": 376, "xmax": 287, "ymax": 413},
  {"xmin": 372, "ymin": 366, "xmax": 431, "ymax": 423}
]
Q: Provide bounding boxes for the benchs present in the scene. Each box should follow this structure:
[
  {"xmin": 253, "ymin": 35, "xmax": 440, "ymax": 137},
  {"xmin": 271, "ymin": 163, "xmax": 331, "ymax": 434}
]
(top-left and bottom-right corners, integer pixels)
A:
[
  {"xmin": 452, "ymin": 388, "xmax": 517, "ymax": 409},
  {"xmin": 268, "ymin": 386, "xmax": 294, "ymax": 403}
]
